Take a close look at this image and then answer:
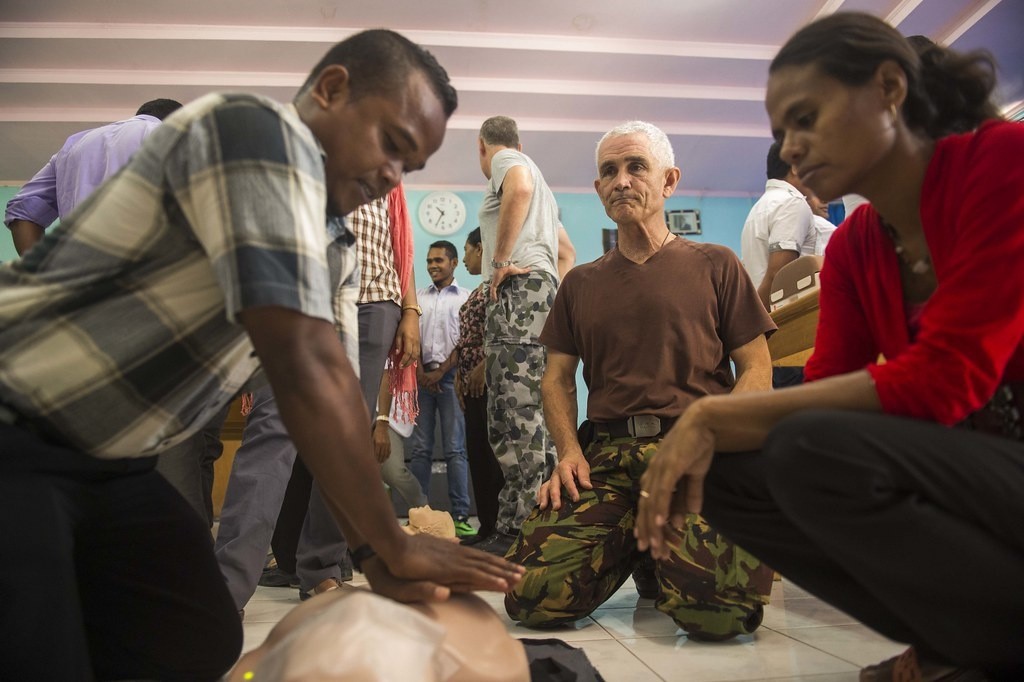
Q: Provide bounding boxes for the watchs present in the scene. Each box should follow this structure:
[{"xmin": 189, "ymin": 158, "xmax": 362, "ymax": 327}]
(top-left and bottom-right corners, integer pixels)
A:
[
  {"xmin": 401, "ymin": 304, "xmax": 423, "ymax": 316},
  {"xmin": 491, "ymin": 258, "xmax": 510, "ymax": 268}
]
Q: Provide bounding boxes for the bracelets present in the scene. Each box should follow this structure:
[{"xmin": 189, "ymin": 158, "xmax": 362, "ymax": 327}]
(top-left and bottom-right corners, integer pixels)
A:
[{"xmin": 376, "ymin": 415, "xmax": 389, "ymax": 423}]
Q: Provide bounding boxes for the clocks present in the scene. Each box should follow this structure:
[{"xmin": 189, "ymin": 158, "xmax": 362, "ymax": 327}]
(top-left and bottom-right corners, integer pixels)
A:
[{"xmin": 418, "ymin": 190, "xmax": 466, "ymax": 236}]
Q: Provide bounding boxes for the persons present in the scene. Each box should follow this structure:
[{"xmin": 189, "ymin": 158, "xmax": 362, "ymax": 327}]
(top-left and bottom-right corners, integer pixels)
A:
[
  {"xmin": 213, "ymin": 173, "xmax": 424, "ymax": 619},
  {"xmin": 632, "ymin": 12, "xmax": 1024, "ymax": 682},
  {"xmin": 408, "ymin": 241, "xmax": 479, "ymax": 537},
  {"xmin": 740, "ymin": 138, "xmax": 840, "ymax": 311},
  {"xmin": 369, "ymin": 341, "xmax": 428, "ymax": 528},
  {"xmin": 502, "ymin": 122, "xmax": 777, "ymax": 641},
  {"xmin": 452, "ymin": 228, "xmax": 501, "ymax": 549},
  {"xmin": 4, "ymin": 99, "xmax": 182, "ymax": 256},
  {"xmin": 223, "ymin": 581, "xmax": 535, "ymax": 682},
  {"xmin": 0, "ymin": 35, "xmax": 525, "ymax": 682},
  {"xmin": 470, "ymin": 116, "xmax": 577, "ymax": 556}
]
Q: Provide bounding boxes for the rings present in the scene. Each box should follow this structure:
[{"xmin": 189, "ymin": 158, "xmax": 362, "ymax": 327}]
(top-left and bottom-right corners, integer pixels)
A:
[{"xmin": 639, "ymin": 490, "xmax": 650, "ymax": 497}]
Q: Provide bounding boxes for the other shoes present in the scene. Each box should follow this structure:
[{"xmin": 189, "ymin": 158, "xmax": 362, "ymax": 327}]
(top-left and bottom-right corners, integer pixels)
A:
[
  {"xmin": 339, "ymin": 551, "xmax": 354, "ymax": 581},
  {"xmin": 470, "ymin": 532, "xmax": 517, "ymax": 553},
  {"xmin": 453, "ymin": 520, "xmax": 479, "ymax": 537},
  {"xmin": 634, "ymin": 561, "xmax": 662, "ymax": 599},
  {"xmin": 297, "ymin": 577, "xmax": 352, "ymax": 600},
  {"xmin": 459, "ymin": 524, "xmax": 498, "ymax": 546},
  {"xmin": 258, "ymin": 563, "xmax": 303, "ymax": 588},
  {"xmin": 862, "ymin": 645, "xmax": 968, "ymax": 682}
]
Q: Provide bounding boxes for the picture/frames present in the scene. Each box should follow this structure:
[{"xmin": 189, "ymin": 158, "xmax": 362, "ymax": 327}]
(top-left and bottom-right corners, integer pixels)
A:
[{"xmin": 665, "ymin": 209, "xmax": 701, "ymax": 236}]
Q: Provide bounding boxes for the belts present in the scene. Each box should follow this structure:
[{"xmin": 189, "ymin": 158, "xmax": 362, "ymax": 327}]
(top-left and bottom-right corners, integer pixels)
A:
[
  {"xmin": 423, "ymin": 362, "xmax": 443, "ymax": 372},
  {"xmin": 592, "ymin": 413, "xmax": 674, "ymax": 438}
]
